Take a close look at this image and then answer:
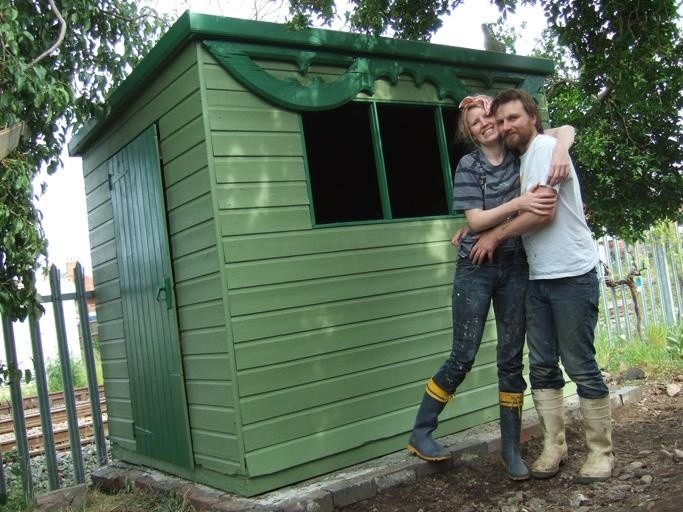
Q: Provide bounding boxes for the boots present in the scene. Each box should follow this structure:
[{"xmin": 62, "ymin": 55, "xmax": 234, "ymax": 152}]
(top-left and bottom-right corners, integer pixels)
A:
[
  {"xmin": 498, "ymin": 393, "xmax": 529, "ymax": 480},
  {"xmin": 406, "ymin": 377, "xmax": 453, "ymax": 462},
  {"xmin": 575, "ymin": 394, "xmax": 614, "ymax": 484},
  {"xmin": 530, "ymin": 388, "xmax": 569, "ymax": 478}
]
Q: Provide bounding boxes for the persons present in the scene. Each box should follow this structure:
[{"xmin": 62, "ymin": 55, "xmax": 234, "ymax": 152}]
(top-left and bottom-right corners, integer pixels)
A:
[
  {"xmin": 409, "ymin": 95, "xmax": 577, "ymax": 480},
  {"xmin": 452, "ymin": 89, "xmax": 615, "ymax": 480}
]
